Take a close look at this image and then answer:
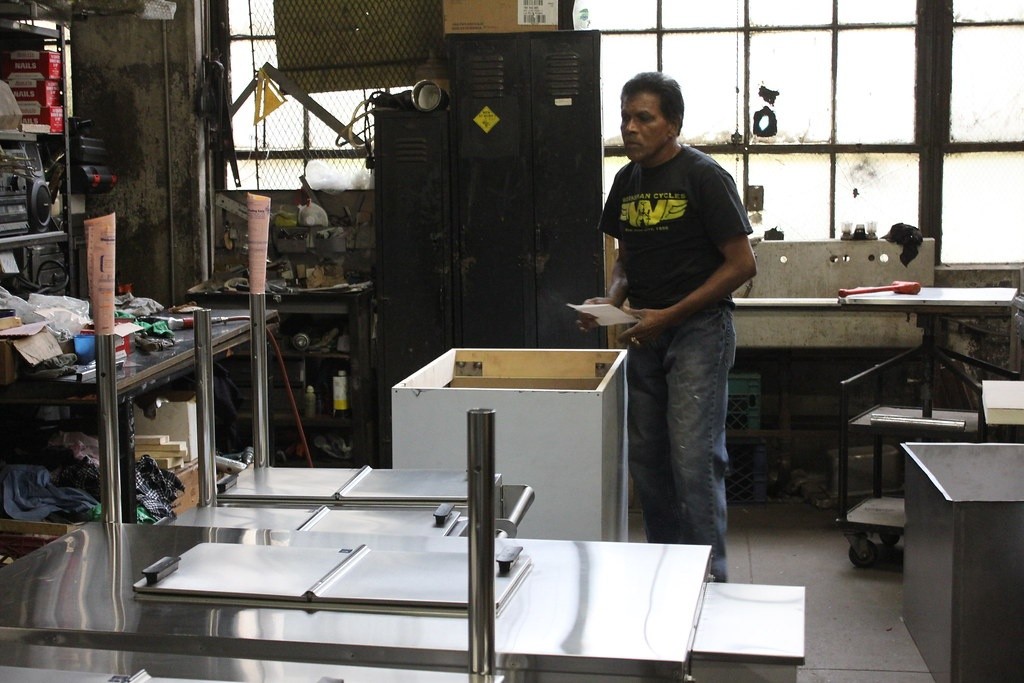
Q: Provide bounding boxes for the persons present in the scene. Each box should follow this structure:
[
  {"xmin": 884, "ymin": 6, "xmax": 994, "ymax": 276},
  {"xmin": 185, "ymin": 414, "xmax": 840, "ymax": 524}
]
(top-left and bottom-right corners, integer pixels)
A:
[{"xmin": 576, "ymin": 72, "xmax": 757, "ymax": 582}]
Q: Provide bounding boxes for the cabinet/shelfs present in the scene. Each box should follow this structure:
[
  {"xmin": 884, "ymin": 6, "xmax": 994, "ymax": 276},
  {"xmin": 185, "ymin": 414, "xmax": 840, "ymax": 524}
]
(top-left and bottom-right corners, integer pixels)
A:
[
  {"xmin": 0, "ymin": 18, "xmax": 78, "ymax": 298},
  {"xmin": 185, "ymin": 279, "xmax": 377, "ymax": 469},
  {"xmin": 445, "ymin": 30, "xmax": 608, "ymax": 349},
  {"xmin": 371, "ymin": 110, "xmax": 454, "ymax": 464}
]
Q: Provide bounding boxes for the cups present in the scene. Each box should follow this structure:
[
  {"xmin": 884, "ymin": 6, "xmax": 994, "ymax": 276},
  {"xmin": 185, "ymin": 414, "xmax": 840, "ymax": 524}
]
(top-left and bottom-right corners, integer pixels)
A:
[
  {"xmin": 75, "ymin": 334, "xmax": 97, "ymax": 365},
  {"xmin": 412, "ymin": 79, "xmax": 449, "ymax": 111}
]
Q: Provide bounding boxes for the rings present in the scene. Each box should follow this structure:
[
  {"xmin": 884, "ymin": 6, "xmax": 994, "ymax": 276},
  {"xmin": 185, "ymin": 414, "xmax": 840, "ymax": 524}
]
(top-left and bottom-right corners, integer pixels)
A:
[{"xmin": 631, "ymin": 337, "xmax": 640, "ymax": 345}]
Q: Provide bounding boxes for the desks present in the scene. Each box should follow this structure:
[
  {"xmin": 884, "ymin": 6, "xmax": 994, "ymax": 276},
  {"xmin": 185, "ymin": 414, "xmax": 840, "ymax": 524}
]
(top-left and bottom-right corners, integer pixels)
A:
[
  {"xmin": 0, "ymin": 310, "xmax": 282, "ymax": 524},
  {"xmin": 837, "ymin": 285, "xmax": 1020, "ymax": 424}
]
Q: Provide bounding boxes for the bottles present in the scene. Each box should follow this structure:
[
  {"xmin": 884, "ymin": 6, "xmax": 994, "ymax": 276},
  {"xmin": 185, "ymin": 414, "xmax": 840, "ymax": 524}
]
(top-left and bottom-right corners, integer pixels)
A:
[
  {"xmin": 304, "ymin": 385, "xmax": 315, "ymax": 418},
  {"xmin": 332, "ymin": 370, "xmax": 351, "ymax": 418}
]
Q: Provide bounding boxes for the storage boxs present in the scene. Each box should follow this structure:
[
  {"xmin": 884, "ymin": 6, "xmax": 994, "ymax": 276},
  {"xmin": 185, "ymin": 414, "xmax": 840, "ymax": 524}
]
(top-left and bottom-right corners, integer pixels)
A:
[
  {"xmin": 20, "ymin": 105, "xmax": 64, "ymax": 133},
  {"xmin": 900, "ymin": 441, "xmax": 1024, "ymax": 683},
  {"xmin": 443, "ymin": 0, "xmax": 559, "ymax": 34},
  {"xmin": 0, "ymin": 329, "xmax": 63, "ymax": 386},
  {"xmin": 80, "ymin": 318, "xmax": 145, "ymax": 360},
  {"xmin": 727, "ymin": 373, "xmax": 762, "ymax": 431},
  {"xmin": 134, "ymin": 392, "xmax": 199, "ymax": 462},
  {"xmin": 4, "ymin": 79, "xmax": 61, "ymax": 108},
  {"xmin": 726, "ymin": 437, "xmax": 767, "ymax": 506},
  {"xmin": 817, "ymin": 444, "xmax": 904, "ymax": 500},
  {"xmin": 0, "ymin": 49, "xmax": 61, "ymax": 81}
]
